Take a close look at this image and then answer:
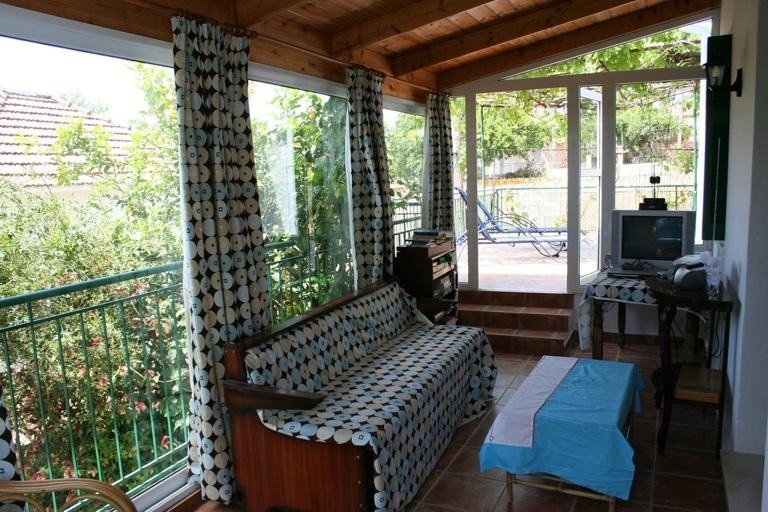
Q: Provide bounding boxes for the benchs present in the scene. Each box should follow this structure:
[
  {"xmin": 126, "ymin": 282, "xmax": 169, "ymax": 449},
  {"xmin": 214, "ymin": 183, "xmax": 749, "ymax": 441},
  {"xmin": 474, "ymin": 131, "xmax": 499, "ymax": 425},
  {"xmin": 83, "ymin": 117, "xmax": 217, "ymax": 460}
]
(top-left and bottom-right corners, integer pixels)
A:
[{"xmin": 218, "ymin": 276, "xmax": 498, "ymax": 511}]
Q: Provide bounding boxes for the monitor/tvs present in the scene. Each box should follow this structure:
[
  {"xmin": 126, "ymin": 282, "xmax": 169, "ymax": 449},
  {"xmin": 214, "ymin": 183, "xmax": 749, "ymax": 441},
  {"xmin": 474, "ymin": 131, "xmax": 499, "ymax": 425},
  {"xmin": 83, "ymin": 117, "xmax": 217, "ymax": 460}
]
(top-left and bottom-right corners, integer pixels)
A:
[{"xmin": 610, "ymin": 208, "xmax": 696, "ymax": 271}]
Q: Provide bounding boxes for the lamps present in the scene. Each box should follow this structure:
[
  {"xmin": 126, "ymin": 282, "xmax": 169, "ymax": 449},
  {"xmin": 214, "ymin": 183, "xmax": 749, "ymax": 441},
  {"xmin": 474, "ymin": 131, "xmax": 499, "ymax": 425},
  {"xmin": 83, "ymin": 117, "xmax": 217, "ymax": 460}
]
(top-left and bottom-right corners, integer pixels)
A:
[{"xmin": 702, "ymin": 53, "xmax": 744, "ymax": 99}]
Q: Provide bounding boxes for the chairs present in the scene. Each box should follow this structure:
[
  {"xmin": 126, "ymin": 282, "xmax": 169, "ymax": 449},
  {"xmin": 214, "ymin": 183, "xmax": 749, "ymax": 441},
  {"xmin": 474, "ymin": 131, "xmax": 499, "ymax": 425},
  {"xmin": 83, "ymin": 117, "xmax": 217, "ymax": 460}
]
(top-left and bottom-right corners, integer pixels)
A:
[{"xmin": 454, "ymin": 186, "xmax": 589, "ymax": 264}]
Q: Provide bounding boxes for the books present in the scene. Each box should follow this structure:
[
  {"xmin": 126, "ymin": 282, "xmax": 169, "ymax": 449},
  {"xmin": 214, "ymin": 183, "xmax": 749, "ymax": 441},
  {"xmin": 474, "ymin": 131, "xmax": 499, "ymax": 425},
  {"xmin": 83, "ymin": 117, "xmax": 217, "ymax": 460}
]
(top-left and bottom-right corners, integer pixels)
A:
[{"xmin": 407, "ymin": 230, "xmax": 455, "ymax": 321}]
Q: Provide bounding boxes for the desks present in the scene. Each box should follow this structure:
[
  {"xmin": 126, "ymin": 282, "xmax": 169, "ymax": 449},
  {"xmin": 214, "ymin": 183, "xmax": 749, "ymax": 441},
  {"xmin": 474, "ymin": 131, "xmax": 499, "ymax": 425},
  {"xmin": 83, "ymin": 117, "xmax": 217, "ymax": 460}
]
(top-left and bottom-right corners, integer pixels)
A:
[{"xmin": 576, "ymin": 268, "xmax": 723, "ymax": 368}]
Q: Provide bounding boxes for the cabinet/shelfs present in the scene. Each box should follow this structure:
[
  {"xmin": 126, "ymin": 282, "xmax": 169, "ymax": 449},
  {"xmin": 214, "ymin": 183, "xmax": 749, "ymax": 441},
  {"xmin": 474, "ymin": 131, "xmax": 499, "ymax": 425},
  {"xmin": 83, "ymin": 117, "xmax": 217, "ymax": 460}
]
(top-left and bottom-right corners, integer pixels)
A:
[
  {"xmin": 395, "ymin": 231, "xmax": 458, "ymax": 327},
  {"xmin": 645, "ymin": 271, "xmax": 736, "ymax": 463}
]
